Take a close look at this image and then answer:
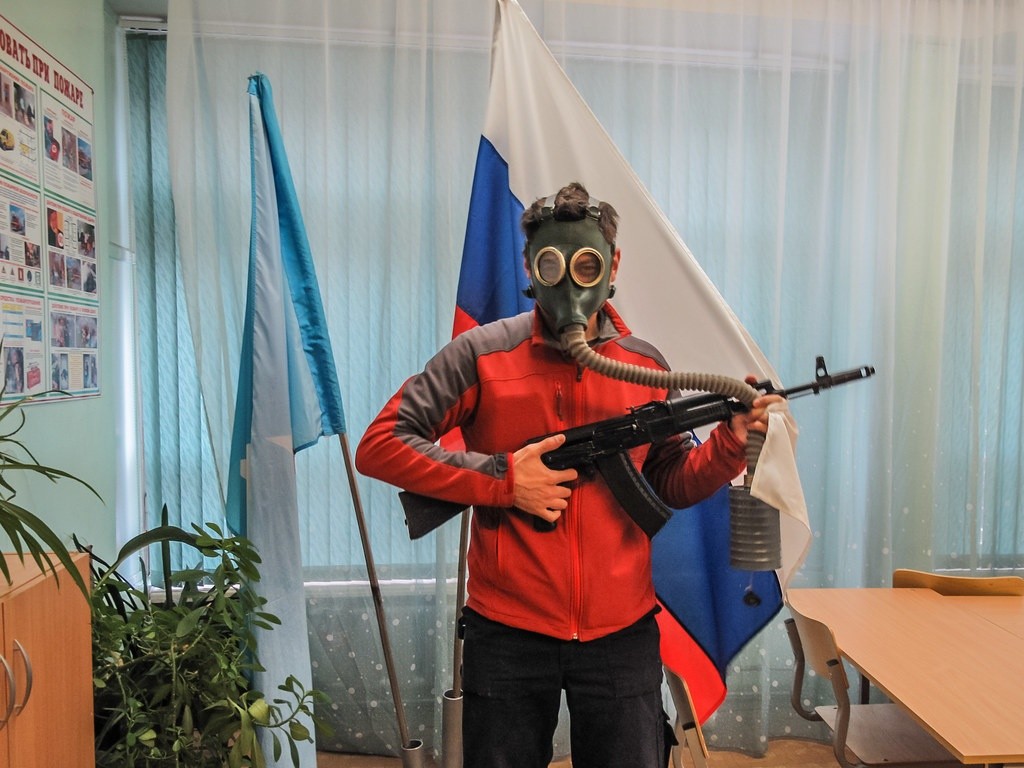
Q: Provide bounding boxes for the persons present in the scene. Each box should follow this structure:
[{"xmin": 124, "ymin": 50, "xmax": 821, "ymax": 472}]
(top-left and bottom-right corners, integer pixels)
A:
[
  {"xmin": 48, "ymin": 208, "xmax": 64, "ymax": 250},
  {"xmin": 356, "ymin": 182, "xmax": 788, "ymax": 768}
]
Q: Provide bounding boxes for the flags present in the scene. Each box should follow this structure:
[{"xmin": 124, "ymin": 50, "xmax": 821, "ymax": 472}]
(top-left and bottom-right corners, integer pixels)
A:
[
  {"xmin": 452, "ymin": 0, "xmax": 813, "ymax": 729},
  {"xmin": 222, "ymin": 74, "xmax": 347, "ymax": 768}
]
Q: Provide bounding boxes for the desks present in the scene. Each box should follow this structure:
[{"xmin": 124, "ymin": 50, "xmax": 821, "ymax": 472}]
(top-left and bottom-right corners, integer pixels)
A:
[
  {"xmin": 943, "ymin": 594, "xmax": 1023, "ymax": 638},
  {"xmin": 784, "ymin": 588, "xmax": 1023, "ymax": 768}
]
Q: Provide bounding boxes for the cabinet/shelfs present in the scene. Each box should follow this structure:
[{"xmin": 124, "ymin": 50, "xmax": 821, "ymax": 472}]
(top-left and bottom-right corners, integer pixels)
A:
[{"xmin": 0, "ymin": 552, "xmax": 97, "ymax": 767}]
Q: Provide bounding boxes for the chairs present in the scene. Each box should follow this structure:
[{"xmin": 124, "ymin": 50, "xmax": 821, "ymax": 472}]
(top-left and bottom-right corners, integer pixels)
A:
[
  {"xmin": 784, "ymin": 599, "xmax": 988, "ymax": 768},
  {"xmin": 892, "ymin": 569, "xmax": 1023, "ymax": 597},
  {"xmin": 663, "ymin": 665, "xmax": 709, "ymax": 768}
]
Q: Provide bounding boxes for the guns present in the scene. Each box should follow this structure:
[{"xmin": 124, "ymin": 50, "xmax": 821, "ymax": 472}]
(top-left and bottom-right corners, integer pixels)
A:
[{"xmin": 398, "ymin": 354, "xmax": 876, "ymax": 542}]
